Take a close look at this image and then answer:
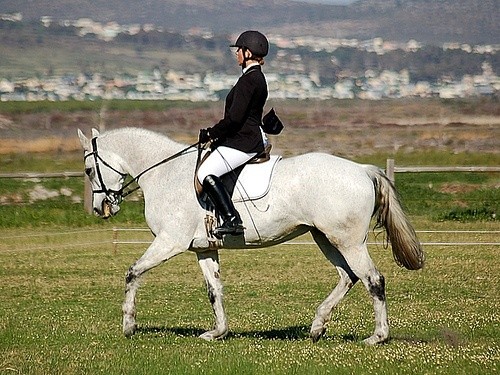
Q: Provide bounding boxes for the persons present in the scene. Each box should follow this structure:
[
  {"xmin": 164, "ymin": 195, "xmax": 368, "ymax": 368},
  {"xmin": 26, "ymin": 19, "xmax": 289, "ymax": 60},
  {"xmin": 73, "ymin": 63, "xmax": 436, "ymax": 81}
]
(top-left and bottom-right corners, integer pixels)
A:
[{"xmin": 197, "ymin": 30, "xmax": 269, "ymax": 233}]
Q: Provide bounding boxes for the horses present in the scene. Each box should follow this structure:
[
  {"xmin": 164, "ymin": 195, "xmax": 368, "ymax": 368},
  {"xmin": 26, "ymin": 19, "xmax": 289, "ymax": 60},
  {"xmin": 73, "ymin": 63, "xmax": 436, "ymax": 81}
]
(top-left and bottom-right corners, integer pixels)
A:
[{"xmin": 77, "ymin": 128, "xmax": 426, "ymax": 344}]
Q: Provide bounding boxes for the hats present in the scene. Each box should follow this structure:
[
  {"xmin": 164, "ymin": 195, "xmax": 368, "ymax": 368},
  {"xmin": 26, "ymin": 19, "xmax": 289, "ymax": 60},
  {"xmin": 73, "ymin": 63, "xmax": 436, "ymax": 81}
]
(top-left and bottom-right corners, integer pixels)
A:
[{"xmin": 229, "ymin": 31, "xmax": 269, "ymax": 57}]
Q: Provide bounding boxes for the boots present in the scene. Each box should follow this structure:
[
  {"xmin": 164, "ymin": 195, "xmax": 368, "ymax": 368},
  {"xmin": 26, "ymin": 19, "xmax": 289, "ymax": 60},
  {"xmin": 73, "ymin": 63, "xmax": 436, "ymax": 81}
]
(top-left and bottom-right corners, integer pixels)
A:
[{"xmin": 200, "ymin": 175, "xmax": 245, "ymax": 235}]
